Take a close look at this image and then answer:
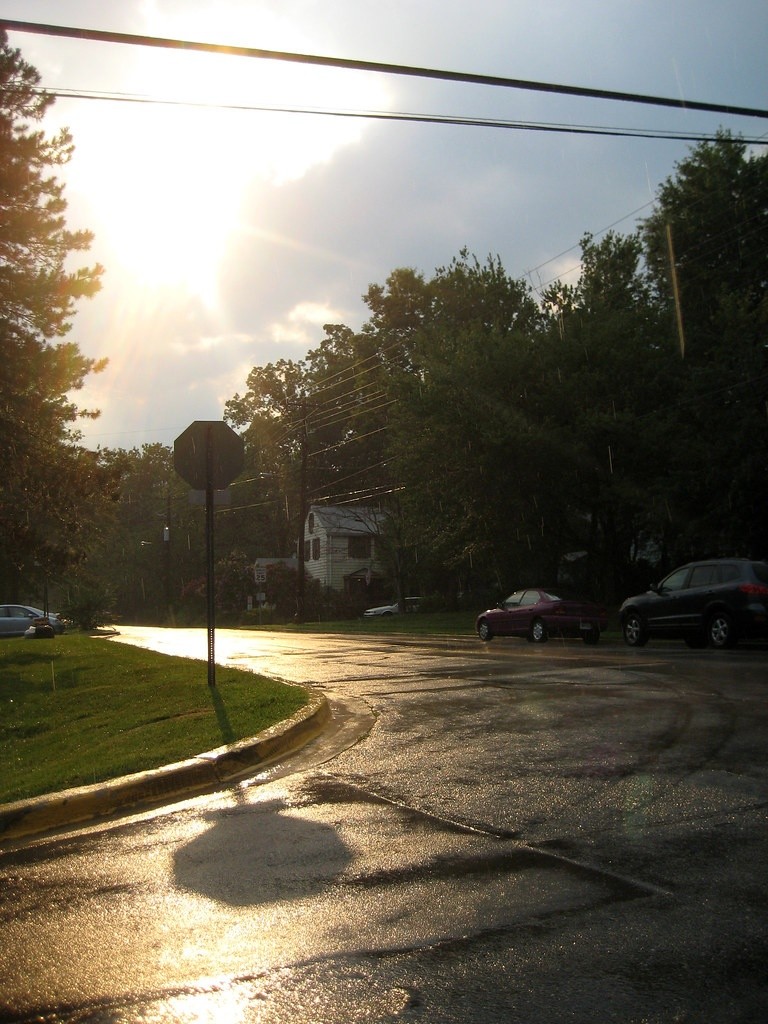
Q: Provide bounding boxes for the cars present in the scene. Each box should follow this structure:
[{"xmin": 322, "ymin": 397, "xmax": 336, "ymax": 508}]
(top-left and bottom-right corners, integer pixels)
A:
[
  {"xmin": 475, "ymin": 585, "xmax": 609, "ymax": 645},
  {"xmin": 363, "ymin": 597, "xmax": 424, "ymax": 619},
  {"xmin": 0, "ymin": 604, "xmax": 64, "ymax": 636}
]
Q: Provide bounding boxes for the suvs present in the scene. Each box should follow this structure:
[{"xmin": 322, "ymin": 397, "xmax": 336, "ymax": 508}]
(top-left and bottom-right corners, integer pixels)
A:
[{"xmin": 615, "ymin": 557, "xmax": 768, "ymax": 651}]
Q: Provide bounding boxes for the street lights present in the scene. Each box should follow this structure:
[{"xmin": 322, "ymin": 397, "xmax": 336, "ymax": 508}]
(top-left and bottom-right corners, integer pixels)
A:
[
  {"xmin": 258, "ymin": 473, "xmax": 304, "ymax": 623},
  {"xmin": 141, "ymin": 539, "xmax": 171, "ymax": 623}
]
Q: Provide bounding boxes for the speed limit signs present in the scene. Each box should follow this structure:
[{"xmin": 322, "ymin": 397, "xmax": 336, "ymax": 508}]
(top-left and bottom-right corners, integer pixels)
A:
[{"xmin": 254, "ymin": 559, "xmax": 266, "ymax": 583}]
